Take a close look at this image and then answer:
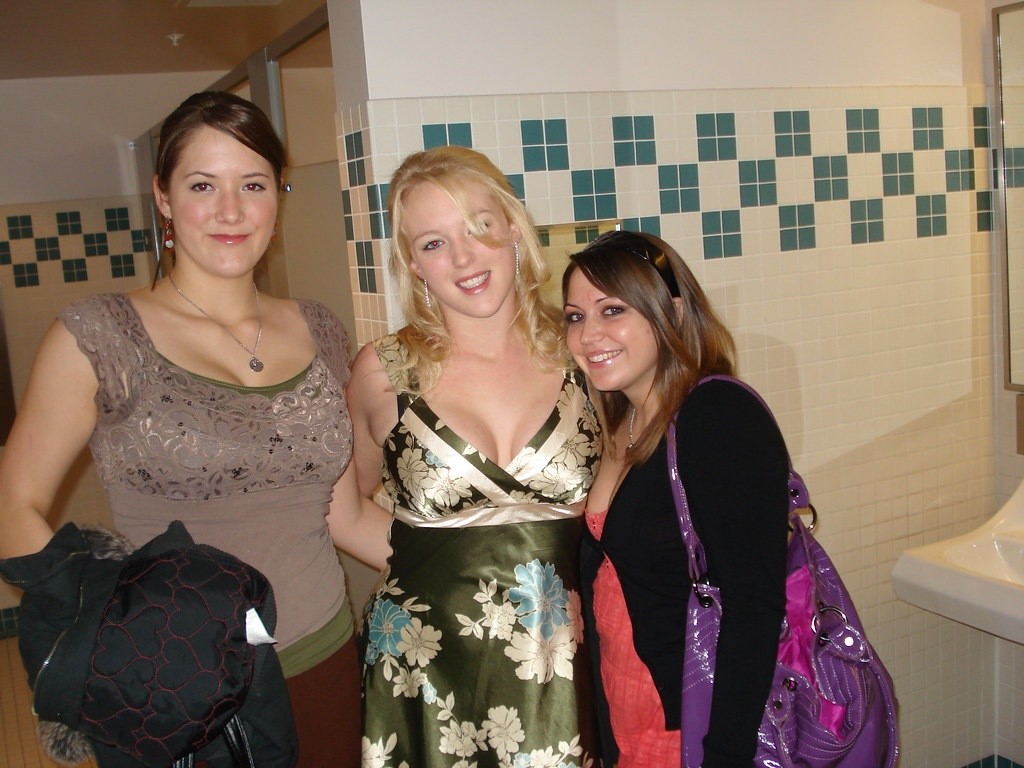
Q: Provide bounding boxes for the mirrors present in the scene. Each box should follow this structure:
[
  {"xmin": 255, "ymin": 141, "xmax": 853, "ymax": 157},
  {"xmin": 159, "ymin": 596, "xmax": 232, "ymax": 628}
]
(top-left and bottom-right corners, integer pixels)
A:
[{"xmin": 993, "ymin": 2, "xmax": 1024, "ymax": 393}]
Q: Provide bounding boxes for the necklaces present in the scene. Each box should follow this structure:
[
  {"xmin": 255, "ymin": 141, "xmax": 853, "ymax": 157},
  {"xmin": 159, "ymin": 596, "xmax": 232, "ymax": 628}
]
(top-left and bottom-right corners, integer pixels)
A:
[
  {"xmin": 168, "ymin": 260, "xmax": 266, "ymax": 372},
  {"xmin": 627, "ymin": 403, "xmax": 637, "ymax": 453}
]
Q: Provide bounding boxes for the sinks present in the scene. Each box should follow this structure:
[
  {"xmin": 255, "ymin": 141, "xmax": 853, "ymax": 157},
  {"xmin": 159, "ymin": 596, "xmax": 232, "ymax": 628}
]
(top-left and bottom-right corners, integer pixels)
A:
[{"xmin": 891, "ymin": 476, "xmax": 1024, "ymax": 645}]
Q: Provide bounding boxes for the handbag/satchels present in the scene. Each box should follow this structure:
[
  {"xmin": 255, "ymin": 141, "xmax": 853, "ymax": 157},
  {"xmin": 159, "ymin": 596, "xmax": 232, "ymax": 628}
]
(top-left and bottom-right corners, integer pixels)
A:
[{"xmin": 667, "ymin": 377, "xmax": 900, "ymax": 768}]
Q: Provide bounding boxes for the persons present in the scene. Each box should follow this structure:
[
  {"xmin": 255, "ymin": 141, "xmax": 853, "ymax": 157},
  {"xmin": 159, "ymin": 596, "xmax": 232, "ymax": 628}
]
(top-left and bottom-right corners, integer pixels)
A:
[
  {"xmin": 560, "ymin": 230, "xmax": 790, "ymax": 768},
  {"xmin": 0, "ymin": 92, "xmax": 393, "ymax": 768},
  {"xmin": 347, "ymin": 144, "xmax": 643, "ymax": 768}
]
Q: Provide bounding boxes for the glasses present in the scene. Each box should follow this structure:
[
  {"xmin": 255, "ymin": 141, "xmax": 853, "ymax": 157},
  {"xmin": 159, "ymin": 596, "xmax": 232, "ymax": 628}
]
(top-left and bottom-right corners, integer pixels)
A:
[{"xmin": 586, "ymin": 232, "xmax": 681, "ymax": 298}]
